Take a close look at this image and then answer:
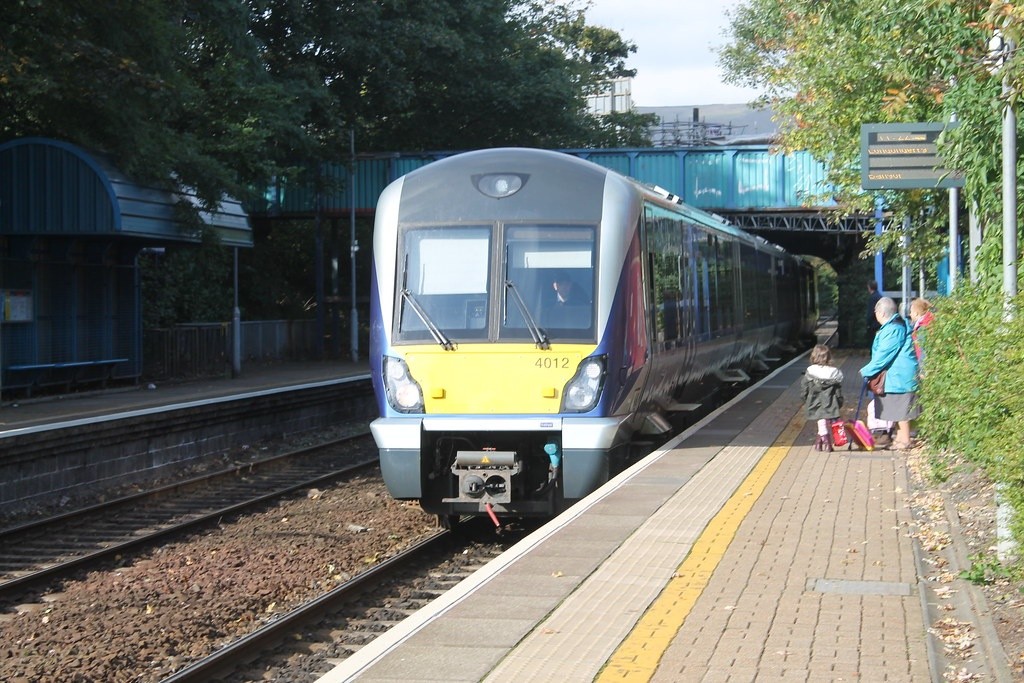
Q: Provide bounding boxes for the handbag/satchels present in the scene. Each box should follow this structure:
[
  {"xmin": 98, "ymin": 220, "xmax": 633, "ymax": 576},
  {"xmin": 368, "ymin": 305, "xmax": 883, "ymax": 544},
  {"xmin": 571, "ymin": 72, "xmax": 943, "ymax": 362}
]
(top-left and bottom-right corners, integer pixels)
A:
[
  {"xmin": 868, "ymin": 370, "xmax": 886, "ymax": 395},
  {"xmin": 831, "ymin": 418, "xmax": 848, "ymax": 446}
]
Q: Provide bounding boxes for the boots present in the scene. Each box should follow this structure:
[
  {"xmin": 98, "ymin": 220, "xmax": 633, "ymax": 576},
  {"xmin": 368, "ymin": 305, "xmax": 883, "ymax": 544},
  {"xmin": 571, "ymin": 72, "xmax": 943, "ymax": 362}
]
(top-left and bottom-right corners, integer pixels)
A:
[
  {"xmin": 815, "ymin": 434, "xmax": 822, "ymax": 451},
  {"xmin": 876, "ymin": 430, "xmax": 893, "ymax": 446},
  {"xmin": 821, "ymin": 434, "xmax": 833, "ymax": 452}
]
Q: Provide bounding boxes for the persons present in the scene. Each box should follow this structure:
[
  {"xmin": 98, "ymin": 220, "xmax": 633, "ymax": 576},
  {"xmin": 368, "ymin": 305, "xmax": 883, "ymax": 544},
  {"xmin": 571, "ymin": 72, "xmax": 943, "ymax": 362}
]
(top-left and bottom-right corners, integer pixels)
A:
[
  {"xmin": 540, "ymin": 270, "xmax": 589, "ymax": 328},
  {"xmin": 864, "ymin": 281, "xmax": 883, "ymax": 355},
  {"xmin": 800, "ymin": 344, "xmax": 844, "ymax": 452},
  {"xmin": 859, "ymin": 296, "xmax": 921, "ymax": 450},
  {"xmin": 909, "ymin": 298, "xmax": 935, "ymax": 367}
]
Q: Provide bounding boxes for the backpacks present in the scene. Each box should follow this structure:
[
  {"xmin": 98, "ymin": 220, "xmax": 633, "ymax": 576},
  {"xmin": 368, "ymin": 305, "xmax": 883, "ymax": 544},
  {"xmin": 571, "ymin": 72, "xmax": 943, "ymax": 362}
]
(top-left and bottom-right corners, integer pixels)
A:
[{"xmin": 845, "ymin": 419, "xmax": 875, "ymax": 451}]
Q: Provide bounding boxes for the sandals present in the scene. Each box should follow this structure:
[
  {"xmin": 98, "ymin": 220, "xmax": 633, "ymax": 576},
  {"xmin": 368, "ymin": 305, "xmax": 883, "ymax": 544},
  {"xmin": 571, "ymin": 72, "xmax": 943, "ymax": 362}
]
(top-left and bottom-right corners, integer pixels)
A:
[{"xmin": 884, "ymin": 442, "xmax": 913, "ymax": 450}]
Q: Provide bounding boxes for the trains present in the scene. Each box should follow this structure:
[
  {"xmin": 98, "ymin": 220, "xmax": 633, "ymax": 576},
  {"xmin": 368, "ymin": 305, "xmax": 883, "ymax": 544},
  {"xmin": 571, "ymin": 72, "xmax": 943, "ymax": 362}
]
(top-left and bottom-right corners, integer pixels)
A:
[{"xmin": 363, "ymin": 147, "xmax": 823, "ymax": 538}]
